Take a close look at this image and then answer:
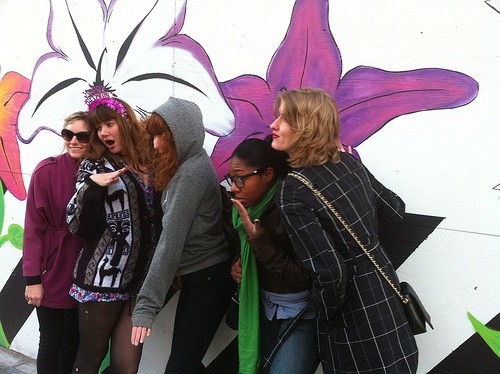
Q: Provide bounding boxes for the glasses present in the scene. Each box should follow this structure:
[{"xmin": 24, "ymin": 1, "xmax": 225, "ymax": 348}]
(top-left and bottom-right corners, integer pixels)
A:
[
  {"xmin": 224, "ymin": 165, "xmax": 271, "ymax": 188},
  {"xmin": 61, "ymin": 129, "xmax": 91, "ymax": 143}
]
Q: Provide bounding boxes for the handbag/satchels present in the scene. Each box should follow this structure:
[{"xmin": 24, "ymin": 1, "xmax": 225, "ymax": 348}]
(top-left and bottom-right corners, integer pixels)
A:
[
  {"xmin": 220, "ymin": 184, "xmax": 240, "ymax": 266},
  {"xmin": 400, "ymin": 282, "xmax": 434, "ymax": 335},
  {"xmin": 225, "ymin": 292, "xmax": 239, "ymax": 330}
]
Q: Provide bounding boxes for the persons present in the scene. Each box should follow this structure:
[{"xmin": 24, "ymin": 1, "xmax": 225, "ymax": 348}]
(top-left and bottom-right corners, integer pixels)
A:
[
  {"xmin": 131, "ymin": 98, "xmax": 240, "ymax": 374},
  {"xmin": 67, "ymin": 99, "xmax": 165, "ymax": 373},
  {"xmin": 23, "ymin": 112, "xmax": 105, "ymax": 374},
  {"xmin": 225, "ymin": 131, "xmax": 325, "ymax": 374},
  {"xmin": 270, "ymin": 87, "xmax": 419, "ymax": 374}
]
